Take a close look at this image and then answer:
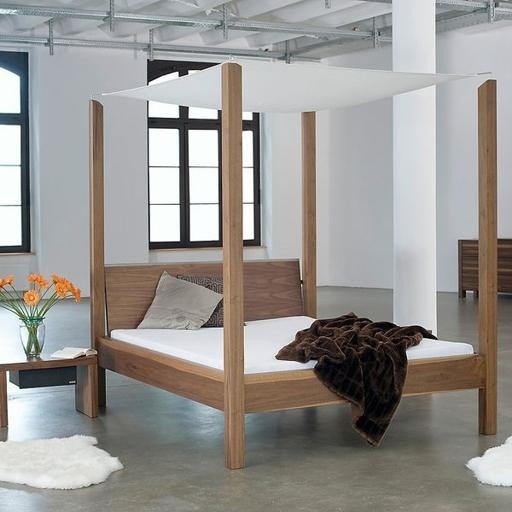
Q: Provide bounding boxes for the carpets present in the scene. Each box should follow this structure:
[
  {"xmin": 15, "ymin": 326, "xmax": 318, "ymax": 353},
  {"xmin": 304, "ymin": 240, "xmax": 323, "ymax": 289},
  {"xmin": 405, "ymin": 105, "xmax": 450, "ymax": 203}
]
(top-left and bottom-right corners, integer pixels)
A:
[{"xmin": 1, "ymin": 434, "xmax": 123, "ymax": 490}]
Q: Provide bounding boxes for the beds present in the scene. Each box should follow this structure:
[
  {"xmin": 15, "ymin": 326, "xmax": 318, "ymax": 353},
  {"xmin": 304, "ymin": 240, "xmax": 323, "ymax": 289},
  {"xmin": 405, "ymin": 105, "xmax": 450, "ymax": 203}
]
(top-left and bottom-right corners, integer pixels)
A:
[{"xmin": 100, "ymin": 256, "xmax": 499, "ymax": 472}]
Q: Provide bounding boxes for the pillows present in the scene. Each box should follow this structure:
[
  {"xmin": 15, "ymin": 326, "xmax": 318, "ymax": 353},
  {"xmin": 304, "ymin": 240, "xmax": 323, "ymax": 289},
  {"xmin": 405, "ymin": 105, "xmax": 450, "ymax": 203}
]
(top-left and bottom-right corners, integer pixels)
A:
[{"xmin": 136, "ymin": 270, "xmax": 248, "ymax": 334}]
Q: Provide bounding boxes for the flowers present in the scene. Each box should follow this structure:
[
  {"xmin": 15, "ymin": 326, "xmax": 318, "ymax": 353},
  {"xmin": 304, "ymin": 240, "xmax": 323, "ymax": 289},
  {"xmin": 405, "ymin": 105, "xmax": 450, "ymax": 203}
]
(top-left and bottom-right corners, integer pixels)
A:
[{"xmin": 0, "ymin": 272, "xmax": 83, "ymax": 352}]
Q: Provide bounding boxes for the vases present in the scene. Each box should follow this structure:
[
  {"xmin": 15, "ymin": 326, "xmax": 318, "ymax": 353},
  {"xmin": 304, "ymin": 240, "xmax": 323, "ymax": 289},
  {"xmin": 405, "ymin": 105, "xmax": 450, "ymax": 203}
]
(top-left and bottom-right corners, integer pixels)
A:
[{"xmin": 18, "ymin": 314, "xmax": 48, "ymax": 357}]
